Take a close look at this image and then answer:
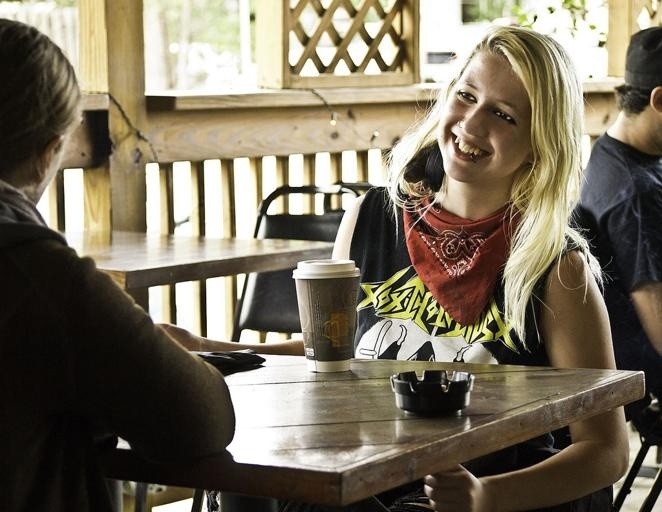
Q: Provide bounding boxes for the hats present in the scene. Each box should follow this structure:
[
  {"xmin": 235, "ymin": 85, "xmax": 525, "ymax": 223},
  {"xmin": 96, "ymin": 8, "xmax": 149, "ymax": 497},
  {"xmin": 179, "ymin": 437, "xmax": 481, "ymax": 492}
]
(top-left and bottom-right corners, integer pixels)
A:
[{"xmin": 624, "ymin": 27, "xmax": 662, "ymax": 88}]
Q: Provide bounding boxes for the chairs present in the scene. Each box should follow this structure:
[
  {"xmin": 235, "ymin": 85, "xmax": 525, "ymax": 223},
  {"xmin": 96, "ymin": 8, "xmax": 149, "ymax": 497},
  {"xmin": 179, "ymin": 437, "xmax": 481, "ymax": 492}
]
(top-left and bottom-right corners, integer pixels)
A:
[{"xmin": 231, "ymin": 186, "xmax": 368, "ymax": 351}]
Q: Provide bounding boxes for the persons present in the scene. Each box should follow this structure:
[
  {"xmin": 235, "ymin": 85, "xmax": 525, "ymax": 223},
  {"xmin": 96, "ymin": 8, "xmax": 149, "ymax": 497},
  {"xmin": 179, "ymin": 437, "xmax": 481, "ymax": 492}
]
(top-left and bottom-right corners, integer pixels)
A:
[
  {"xmin": 570, "ymin": 27, "xmax": 662, "ymax": 447},
  {"xmin": 1, "ymin": 17, "xmax": 236, "ymax": 512},
  {"xmin": 148, "ymin": 28, "xmax": 630, "ymax": 511}
]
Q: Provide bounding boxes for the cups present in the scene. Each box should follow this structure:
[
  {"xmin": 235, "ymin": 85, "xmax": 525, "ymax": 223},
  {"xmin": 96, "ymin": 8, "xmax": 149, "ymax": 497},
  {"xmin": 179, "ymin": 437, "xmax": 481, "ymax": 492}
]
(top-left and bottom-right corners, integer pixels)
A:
[{"xmin": 291, "ymin": 258, "xmax": 361, "ymax": 374}]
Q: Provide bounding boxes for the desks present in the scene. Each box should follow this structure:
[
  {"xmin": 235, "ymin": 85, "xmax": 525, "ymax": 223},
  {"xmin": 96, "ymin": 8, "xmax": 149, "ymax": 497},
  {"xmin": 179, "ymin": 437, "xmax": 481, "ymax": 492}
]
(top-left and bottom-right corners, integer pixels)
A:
[{"xmin": 56, "ymin": 229, "xmax": 336, "ymax": 320}]
